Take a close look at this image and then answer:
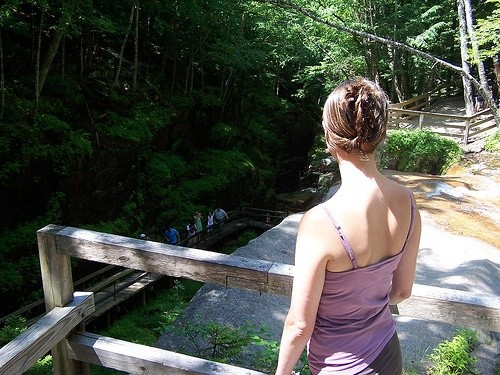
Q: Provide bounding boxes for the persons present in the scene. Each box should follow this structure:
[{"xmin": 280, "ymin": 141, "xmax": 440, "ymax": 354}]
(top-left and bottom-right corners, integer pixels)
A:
[
  {"xmin": 164, "ymin": 225, "xmax": 180, "ymax": 245},
  {"xmin": 206, "ymin": 206, "xmax": 229, "ymax": 234},
  {"xmin": 274, "ymin": 76, "xmax": 423, "ymax": 375},
  {"xmin": 187, "ymin": 211, "xmax": 203, "ymax": 246},
  {"xmin": 140, "ymin": 234, "xmax": 150, "ymax": 241}
]
